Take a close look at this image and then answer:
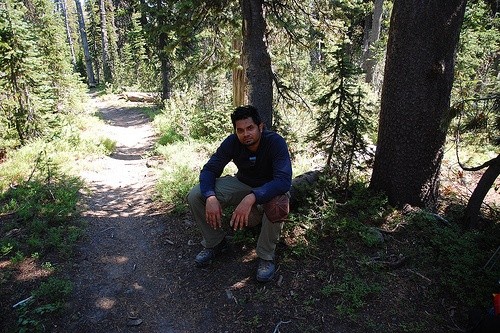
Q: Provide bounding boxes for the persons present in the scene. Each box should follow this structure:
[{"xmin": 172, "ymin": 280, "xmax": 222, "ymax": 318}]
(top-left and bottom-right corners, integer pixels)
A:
[{"xmin": 188, "ymin": 105, "xmax": 292, "ymax": 282}]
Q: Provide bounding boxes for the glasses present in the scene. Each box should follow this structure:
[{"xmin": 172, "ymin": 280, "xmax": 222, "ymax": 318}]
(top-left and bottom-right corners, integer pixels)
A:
[{"xmin": 248, "ymin": 156, "xmax": 256, "ymax": 176}]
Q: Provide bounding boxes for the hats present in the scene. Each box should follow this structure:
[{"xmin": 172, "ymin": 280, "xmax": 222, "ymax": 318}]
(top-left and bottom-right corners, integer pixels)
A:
[{"xmin": 265, "ymin": 193, "xmax": 290, "ymax": 223}]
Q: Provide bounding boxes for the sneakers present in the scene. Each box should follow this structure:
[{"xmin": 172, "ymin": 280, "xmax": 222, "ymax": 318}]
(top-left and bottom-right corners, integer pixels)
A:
[
  {"xmin": 195, "ymin": 238, "xmax": 228, "ymax": 266},
  {"xmin": 257, "ymin": 257, "xmax": 276, "ymax": 282}
]
